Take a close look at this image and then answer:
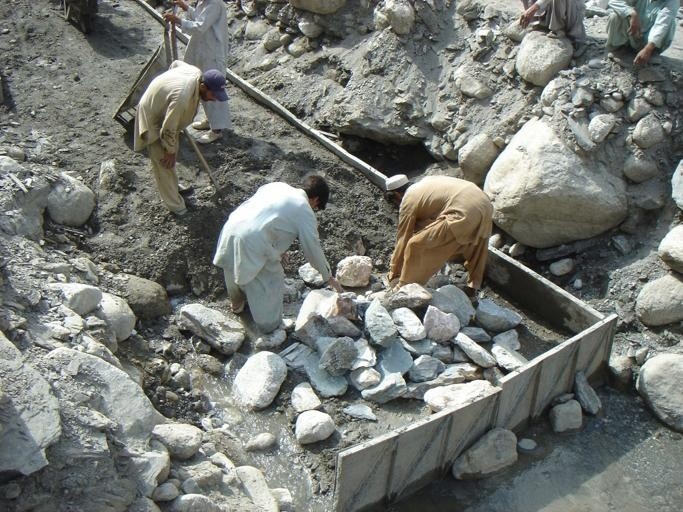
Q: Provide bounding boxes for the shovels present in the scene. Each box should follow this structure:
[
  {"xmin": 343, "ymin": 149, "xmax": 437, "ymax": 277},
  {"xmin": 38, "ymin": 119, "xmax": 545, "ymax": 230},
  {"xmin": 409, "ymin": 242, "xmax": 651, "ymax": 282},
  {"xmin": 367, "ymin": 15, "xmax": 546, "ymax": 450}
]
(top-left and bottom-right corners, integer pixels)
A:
[{"xmin": 184, "ymin": 130, "xmax": 233, "ymax": 204}]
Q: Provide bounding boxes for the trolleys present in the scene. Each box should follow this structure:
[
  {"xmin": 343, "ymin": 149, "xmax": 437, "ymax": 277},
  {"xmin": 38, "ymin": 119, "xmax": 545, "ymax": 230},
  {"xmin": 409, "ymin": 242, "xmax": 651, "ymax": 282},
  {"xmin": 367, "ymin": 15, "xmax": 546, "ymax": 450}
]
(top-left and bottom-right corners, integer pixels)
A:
[
  {"xmin": 107, "ymin": 0, "xmax": 180, "ymax": 139},
  {"xmin": 60, "ymin": 0, "xmax": 99, "ymax": 34}
]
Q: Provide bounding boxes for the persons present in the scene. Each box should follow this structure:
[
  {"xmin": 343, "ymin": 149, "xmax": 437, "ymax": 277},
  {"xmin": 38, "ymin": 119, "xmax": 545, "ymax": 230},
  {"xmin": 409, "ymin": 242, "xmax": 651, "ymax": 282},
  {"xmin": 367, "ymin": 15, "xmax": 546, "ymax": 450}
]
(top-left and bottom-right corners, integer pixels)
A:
[
  {"xmin": 386, "ymin": 173, "xmax": 494, "ymax": 291},
  {"xmin": 132, "ymin": 58, "xmax": 231, "ymax": 217},
  {"xmin": 604, "ymin": 0, "xmax": 681, "ymax": 69},
  {"xmin": 213, "ymin": 176, "xmax": 343, "ymax": 334},
  {"xmin": 520, "ymin": 1, "xmax": 586, "ymax": 47},
  {"xmin": 164, "ymin": 0, "xmax": 233, "ymax": 146}
]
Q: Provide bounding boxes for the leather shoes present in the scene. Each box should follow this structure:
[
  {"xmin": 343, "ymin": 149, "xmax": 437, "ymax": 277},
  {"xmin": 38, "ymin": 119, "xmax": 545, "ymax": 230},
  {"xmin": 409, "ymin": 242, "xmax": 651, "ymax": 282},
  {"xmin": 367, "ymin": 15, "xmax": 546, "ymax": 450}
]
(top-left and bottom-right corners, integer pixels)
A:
[
  {"xmin": 192, "ymin": 120, "xmax": 210, "ymax": 130},
  {"xmin": 196, "ymin": 130, "xmax": 223, "ymax": 144},
  {"xmin": 178, "ymin": 183, "xmax": 194, "ymax": 194}
]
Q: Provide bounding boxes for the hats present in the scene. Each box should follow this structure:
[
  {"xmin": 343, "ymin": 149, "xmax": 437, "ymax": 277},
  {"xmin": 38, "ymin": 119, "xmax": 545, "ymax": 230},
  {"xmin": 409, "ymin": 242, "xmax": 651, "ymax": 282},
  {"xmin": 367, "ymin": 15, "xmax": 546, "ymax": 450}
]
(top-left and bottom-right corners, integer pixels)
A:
[
  {"xmin": 201, "ymin": 69, "xmax": 230, "ymax": 102},
  {"xmin": 385, "ymin": 173, "xmax": 409, "ymax": 191}
]
